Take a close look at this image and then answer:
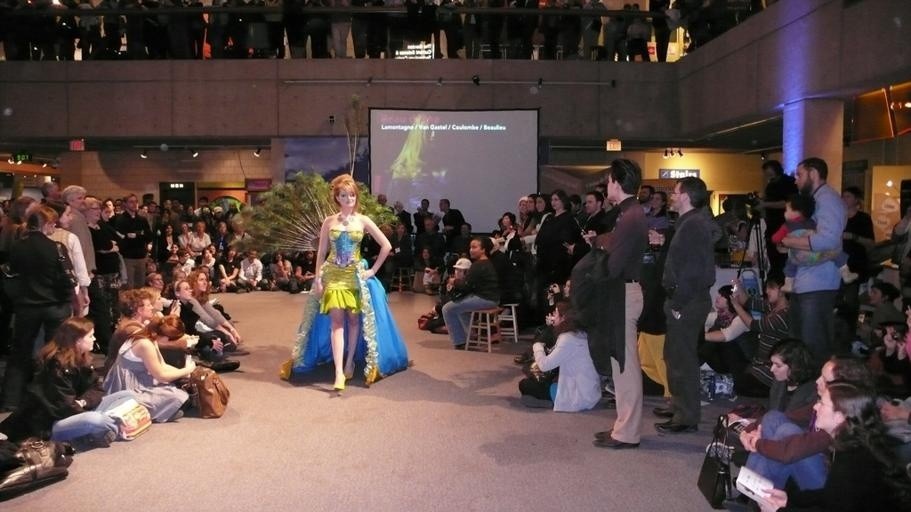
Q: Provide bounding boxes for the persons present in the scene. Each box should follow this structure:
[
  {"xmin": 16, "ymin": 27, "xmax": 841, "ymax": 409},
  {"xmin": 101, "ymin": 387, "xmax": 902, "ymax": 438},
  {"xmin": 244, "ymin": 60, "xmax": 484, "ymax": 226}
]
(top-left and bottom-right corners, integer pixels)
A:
[
  {"xmin": 362, "ymin": 155, "xmax": 911, "ymax": 512},
  {"xmin": 1, "ymin": 0, "xmax": 772, "ymax": 64},
  {"xmin": 310, "ymin": 170, "xmax": 394, "ymax": 396},
  {"xmin": 1, "ymin": 158, "xmax": 321, "ymax": 512}
]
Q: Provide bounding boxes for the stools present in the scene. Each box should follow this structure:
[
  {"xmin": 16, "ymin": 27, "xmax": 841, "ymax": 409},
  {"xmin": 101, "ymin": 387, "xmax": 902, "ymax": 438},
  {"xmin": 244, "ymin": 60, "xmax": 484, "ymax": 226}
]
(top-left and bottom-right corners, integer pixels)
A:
[{"xmin": 464, "ymin": 303, "xmax": 520, "ymax": 353}]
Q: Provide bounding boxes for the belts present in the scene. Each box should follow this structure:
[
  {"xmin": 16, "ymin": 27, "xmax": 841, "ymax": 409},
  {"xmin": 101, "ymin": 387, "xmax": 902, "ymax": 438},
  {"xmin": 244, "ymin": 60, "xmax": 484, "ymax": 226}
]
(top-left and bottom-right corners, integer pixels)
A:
[{"xmin": 624, "ymin": 279, "xmax": 640, "ymax": 283}]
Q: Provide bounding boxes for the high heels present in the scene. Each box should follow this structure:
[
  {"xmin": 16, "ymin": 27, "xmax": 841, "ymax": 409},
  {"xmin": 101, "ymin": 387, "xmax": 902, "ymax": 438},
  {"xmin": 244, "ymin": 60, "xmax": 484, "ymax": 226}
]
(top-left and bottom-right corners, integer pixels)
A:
[
  {"xmin": 344, "ymin": 372, "xmax": 353, "ymax": 380},
  {"xmin": 333, "ymin": 375, "xmax": 346, "ymax": 390}
]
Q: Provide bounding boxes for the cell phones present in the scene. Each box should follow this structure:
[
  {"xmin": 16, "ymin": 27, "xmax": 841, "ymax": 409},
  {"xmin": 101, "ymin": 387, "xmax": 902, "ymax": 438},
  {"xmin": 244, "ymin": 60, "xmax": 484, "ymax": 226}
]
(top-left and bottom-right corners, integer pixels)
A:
[
  {"xmin": 581, "ymin": 228, "xmax": 587, "ymax": 235},
  {"xmin": 176, "ymin": 300, "xmax": 182, "ymax": 307}
]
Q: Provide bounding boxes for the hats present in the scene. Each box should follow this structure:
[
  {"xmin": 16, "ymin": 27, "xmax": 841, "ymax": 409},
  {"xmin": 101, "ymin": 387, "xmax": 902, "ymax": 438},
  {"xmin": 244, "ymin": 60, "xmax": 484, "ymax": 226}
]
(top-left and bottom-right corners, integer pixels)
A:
[
  {"xmin": 519, "ymin": 196, "xmax": 529, "ymax": 206},
  {"xmin": 453, "ymin": 258, "xmax": 472, "ymax": 270}
]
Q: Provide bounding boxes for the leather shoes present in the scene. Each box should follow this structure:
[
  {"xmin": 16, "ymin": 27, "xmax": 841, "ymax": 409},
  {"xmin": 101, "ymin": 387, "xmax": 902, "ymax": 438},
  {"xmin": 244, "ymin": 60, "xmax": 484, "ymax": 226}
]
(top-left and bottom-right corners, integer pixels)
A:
[
  {"xmin": 212, "ymin": 360, "xmax": 240, "ymax": 371},
  {"xmin": 722, "ymin": 493, "xmax": 760, "ymax": 512},
  {"xmin": 595, "ymin": 431, "xmax": 612, "ymax": 438},
  {"xmin": 593, "ymin": 435, "xmax": 641, "ymax": 448},
  {"xmin": 653, "ymin": 407, "xmax": 673, "ymax": 417},
  {"xmin": 655, "ymin": 419, "xmax": 698, "ymax": 432},
  {"xmin": 521, "ymin": 394, "xmax": 554, "ymax": 407}
]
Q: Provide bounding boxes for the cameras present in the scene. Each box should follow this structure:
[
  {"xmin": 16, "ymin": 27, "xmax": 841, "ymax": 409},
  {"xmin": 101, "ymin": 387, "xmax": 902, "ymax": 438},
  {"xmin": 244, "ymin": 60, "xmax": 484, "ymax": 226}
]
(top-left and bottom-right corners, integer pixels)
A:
[
  {"xmin": 747, "ymin": 190, "xmax": 764, "ymax": 220},
  {"xmin": 730, "ymin": 279, "xmax": 738, "ymax": 294},
  {"xmin": 893, "ymin": 330, "xmax": 905, "ymax": 342},
  {"xmin": 546, "ymin": 284, "xmax": 556, "ymax": 296}
]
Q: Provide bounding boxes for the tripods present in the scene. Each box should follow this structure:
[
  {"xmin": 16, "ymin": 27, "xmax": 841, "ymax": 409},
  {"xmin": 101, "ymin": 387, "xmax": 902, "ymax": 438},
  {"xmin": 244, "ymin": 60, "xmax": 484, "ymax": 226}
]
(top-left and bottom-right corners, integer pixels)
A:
[{"xmin": 737, "ymin": 221, "xmax": 767, "ymax": 301}]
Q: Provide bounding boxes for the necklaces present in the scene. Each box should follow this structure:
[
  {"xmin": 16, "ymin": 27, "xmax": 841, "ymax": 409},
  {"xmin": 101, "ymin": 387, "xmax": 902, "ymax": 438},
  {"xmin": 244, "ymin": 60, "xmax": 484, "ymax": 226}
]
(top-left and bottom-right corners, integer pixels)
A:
[{"xmin": 337, "ymin": 210, "xmax": 357, "ymax": 227}]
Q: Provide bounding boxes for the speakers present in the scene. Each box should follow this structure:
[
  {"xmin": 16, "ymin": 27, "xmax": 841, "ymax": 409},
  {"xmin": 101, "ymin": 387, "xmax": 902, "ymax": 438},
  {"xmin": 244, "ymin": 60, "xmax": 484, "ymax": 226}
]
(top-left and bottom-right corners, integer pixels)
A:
[{"xmin": 845, "ymin": 82, "xmax": 911, "ymax": 146}]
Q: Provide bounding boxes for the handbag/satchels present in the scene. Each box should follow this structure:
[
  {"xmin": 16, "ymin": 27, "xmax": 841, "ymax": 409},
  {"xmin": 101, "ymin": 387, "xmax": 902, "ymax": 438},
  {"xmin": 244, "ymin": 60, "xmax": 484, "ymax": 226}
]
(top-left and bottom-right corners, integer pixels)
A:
[
  {"xmin": 700, "ymin": 370, "xmax": 736, "ymax": 401},
  {"xmin": 182, "ymin": 365, "xmax": 230, "ymax": 418},
  {"xmin": 522, "ymin": 351, "xmax": 558, "ymax": 385},
  {"xmin": 698, "ymin": 414, "xmax": 732, "ymax": 509},
  {"xmin": 105, "ymin": 398, "xmax": 153, "ymax": 441},
  {"xmin": 0, "ymin": 430, "xmax": 75, "ymax": 498},
  {"xmin": 287, "ymin": 270, "xmax": 303, "ymax": 294},
  {"xmin": 56, "ymin": 242, "xmax": 76, "ymax": 289},
  {"xmin": 418, "ymin": 312, "xmax": 435, "ymax": 330}
]
